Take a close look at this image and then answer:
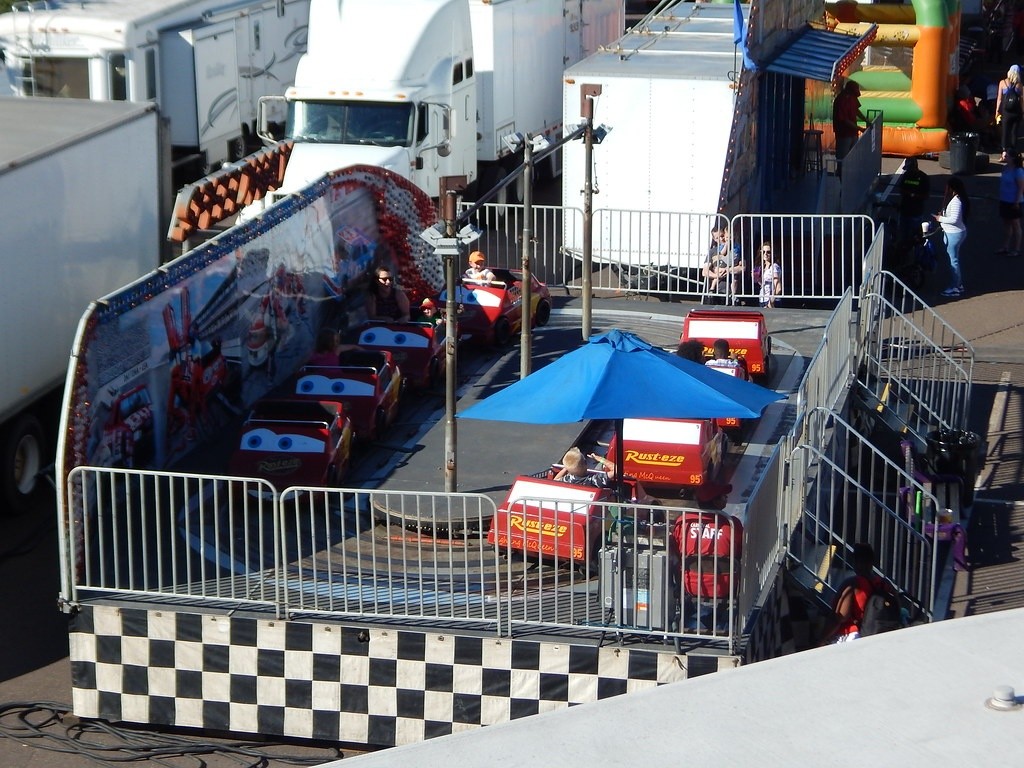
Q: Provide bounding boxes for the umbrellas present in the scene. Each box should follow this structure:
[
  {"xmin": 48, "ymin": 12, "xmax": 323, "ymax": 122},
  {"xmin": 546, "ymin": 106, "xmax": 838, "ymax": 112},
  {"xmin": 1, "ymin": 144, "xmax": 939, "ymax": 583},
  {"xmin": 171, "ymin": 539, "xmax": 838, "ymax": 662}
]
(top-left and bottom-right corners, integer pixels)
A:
[{"xmin": 456, "ymin": 329, "xmax": 789, "ymax": 501}]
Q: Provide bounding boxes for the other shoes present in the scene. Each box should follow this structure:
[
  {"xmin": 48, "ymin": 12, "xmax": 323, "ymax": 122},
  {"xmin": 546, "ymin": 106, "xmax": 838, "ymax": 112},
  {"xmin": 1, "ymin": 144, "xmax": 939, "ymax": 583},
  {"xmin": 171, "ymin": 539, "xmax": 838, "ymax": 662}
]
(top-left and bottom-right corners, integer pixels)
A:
[
  {"xmin": 730, "ymin": 294, "xmax": 740, "ymax": 303},
  {"xmin": 707, "ymin": 289, "xmax": 714, "ymax": 299},
  {"xmin": 1006, "ymin": 250, "xmax": 1022, "ymax": 257},
  {"xmin": 993, "ymin": 247, "xmax": 1009, "ymax": 254},
  {"xmin": 998, "ymin": 156, "xmax": 1006, "ymax": 162}
]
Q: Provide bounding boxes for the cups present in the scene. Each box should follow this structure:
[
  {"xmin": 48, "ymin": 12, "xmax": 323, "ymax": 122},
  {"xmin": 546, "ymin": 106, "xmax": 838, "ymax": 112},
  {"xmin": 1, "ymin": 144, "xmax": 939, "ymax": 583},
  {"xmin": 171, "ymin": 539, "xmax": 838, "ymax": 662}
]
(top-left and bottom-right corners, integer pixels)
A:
[{"xmin": 922, "ymin": 222, "xmax": 929, "ymax": 232}]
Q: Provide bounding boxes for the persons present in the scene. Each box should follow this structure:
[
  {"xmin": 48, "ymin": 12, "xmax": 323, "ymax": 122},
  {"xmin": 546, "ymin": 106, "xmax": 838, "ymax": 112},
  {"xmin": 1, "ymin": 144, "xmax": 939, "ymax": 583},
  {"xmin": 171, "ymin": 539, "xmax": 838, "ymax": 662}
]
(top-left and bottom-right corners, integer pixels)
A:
[
  {"xmin": 701, "ymin": 224, "xmax": 782, "ymax": 309},
  {"xmin": 462, "ymin": 251, "xmax": 496, "ymax": 288},
  {"xmin": 417, "ymin": 298, "xmax": 442, "ymax": 329},
  {"xmin": 833, "ymin": 81, "xmax": 873, "ymax": 181},
  {"xmin": 554, "ymin": 447, "xmax": 619, "ymax": 487},
  {"xmin": 901, "ymin": 0, "xmax": 1024, "ymax": 296},
  {"xmin": 819, "ymin": 544, "xmax": 879, "ymax": 645},
  {"xmin": 296, "ymin": 328, "xmax": 366, "ymax": 378},
  {"xmin": 677, "ymin": 339, "xmax": 739, "ymax": 367},
  {"xmin": 365, "ymin": 265, "xmax": 411, "ymax": 324}
]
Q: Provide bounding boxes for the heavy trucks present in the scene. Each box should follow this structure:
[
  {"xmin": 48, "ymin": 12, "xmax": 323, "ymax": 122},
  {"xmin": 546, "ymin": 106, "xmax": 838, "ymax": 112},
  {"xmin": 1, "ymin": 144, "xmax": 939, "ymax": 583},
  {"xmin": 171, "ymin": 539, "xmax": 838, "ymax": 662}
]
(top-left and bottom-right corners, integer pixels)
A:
[{"xmin": 255, "ymin": 0, "xmax": 630, "ymax": 227}]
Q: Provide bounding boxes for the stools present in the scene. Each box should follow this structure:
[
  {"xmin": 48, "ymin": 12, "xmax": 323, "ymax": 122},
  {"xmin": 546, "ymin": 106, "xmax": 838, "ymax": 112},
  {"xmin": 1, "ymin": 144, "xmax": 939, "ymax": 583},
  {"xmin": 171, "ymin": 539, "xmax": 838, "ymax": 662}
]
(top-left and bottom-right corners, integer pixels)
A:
[{"xmin": 799, "ymin": 130, "xmax": 823, "ymax": 180}]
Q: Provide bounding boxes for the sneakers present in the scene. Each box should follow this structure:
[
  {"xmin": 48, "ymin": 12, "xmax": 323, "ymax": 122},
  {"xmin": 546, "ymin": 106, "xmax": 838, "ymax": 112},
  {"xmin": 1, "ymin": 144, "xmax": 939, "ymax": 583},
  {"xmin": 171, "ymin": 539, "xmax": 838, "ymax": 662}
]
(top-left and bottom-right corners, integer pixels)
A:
[
  {"xmin": 941, "ymin": 287, "xmax": 960, "ymax": 296},
  {"xmin": 959, "ymin": 285, "xmax": 964, "ymax": 292}
]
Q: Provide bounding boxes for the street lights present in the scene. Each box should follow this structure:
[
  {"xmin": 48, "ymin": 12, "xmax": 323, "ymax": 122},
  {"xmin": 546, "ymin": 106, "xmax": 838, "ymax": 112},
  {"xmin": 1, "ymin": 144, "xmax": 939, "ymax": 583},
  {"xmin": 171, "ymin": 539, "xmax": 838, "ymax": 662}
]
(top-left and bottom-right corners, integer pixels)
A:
[
  {"xmin": 416, "ymin": 186, "xmax": 490, "ymax": 499},
  {"xmin": 498, "ymin": 129, "xmax": 550, "ymax": 379},
  {"xmin": 565, "ymin": 94, "xmax": 614, "ymax": 346}
]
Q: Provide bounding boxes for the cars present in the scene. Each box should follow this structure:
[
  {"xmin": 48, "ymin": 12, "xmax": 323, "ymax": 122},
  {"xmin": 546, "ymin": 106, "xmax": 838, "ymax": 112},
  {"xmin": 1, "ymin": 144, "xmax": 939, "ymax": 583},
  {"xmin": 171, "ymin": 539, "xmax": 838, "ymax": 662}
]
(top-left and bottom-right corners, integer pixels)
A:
[
  {"xmin": 693, "ymin": 365, "xmax": 761, "ymax": 447},
  {"xmin": 599, "ymin": 416, "xmax": 732, "ymax": 501},
  {"xmin": 677, "ymin": 306, "xmax": 774, "ymax": 386},
  {"xmin": 346, "ymin": 317, "xmax": 470, "ymax": 392},
  {"xmin": 485, "ymin": 463, "xmax": 667, "ymax": 576},
  {"xmin": 286, "ymin": 346, "xmax": 413, "ymax": 444},
  {"xmin": 225, "ymin": 398, "xmax": 358, "ymax": 511},
  {"xmin": 434, "ymin": 268, "xmax": 556, "ymax": 353}
]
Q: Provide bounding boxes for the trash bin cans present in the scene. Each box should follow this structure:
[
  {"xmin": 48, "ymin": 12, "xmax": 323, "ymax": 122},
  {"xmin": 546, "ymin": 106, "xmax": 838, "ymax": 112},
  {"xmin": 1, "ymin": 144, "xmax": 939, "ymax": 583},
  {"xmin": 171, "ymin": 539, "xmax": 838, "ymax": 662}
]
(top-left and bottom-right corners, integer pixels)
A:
[
  {"xmin": 950, "ymin": 132, "xmax": 981, "ymax": 176},
  {"xmin": 925, "ymin": 429, "xmax": 983, "ymax": 507}
]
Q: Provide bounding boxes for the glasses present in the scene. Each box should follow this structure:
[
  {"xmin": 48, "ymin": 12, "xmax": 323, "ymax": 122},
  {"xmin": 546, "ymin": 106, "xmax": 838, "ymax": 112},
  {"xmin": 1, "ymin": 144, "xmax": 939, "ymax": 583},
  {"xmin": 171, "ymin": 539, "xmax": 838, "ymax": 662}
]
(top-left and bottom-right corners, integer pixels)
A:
[
  {"xmin": 378, "ymin": 277, "xmax": 393, "ymax": 282},
  {"xmin": 762, "ymin": 251, "xmax": 771, "ymax": 254}
]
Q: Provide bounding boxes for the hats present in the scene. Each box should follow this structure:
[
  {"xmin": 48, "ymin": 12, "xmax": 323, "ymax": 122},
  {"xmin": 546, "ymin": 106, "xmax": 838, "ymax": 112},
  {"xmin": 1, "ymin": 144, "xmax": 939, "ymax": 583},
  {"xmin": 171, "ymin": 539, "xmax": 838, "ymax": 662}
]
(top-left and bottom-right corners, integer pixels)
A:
[
  {"xmin": 696, "ymin": 479, "xmax": 733, "ymax": 502},
  {"xmin": 418, "ymin": 297, "xmax": 438, "ymax": 310},
  {"xmin": 845, "ymin": 81, "xmax": 860, "ymax": 97},
  {"xmin": 469, "ymin": 251, "xmax": 485, "ymax": 264},
  {"xmin": 903, "ymin": 158, "xmax": 918, "ymax": 170}
]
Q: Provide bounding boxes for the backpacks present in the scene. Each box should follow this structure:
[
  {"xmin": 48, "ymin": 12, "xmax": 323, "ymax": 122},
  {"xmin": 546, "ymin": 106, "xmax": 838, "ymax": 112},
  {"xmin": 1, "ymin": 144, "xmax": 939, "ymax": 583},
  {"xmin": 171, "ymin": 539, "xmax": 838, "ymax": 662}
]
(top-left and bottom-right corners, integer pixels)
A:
[
  {"xmin": 999, "ymin": 80, "xmax": 1022, "ymax": 121},
  {"xmin": 850, "ymin": 576, "xmax": 901, "ymax": 637}
]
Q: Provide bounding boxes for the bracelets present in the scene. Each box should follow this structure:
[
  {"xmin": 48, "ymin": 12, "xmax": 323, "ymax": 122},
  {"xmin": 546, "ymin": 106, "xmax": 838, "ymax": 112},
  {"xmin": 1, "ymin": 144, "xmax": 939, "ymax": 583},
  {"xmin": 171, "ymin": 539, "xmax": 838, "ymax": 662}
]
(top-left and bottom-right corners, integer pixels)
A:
[{"xmin": 600, "ymin": 457, "xmax": 606, "ymax": 464}]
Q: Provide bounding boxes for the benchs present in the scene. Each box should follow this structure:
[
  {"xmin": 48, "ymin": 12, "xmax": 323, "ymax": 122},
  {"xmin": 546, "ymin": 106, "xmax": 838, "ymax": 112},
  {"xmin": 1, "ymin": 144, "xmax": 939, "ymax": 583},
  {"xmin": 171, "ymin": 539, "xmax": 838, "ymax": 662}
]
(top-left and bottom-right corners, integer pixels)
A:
[
  {"xmin": 259, "ymin": 401, "xmax": 342, "ymax": 430},
  {"xmin": 486, "ymin": 266, "xmax": 523, "ymax": 283},
  {"xmin": 409, "ymin": 307, "xmax": 447, "ymax": 331},
  {"xmin": 339, "ymin": 349, "xmax": 395, "ymax": 378}
]
